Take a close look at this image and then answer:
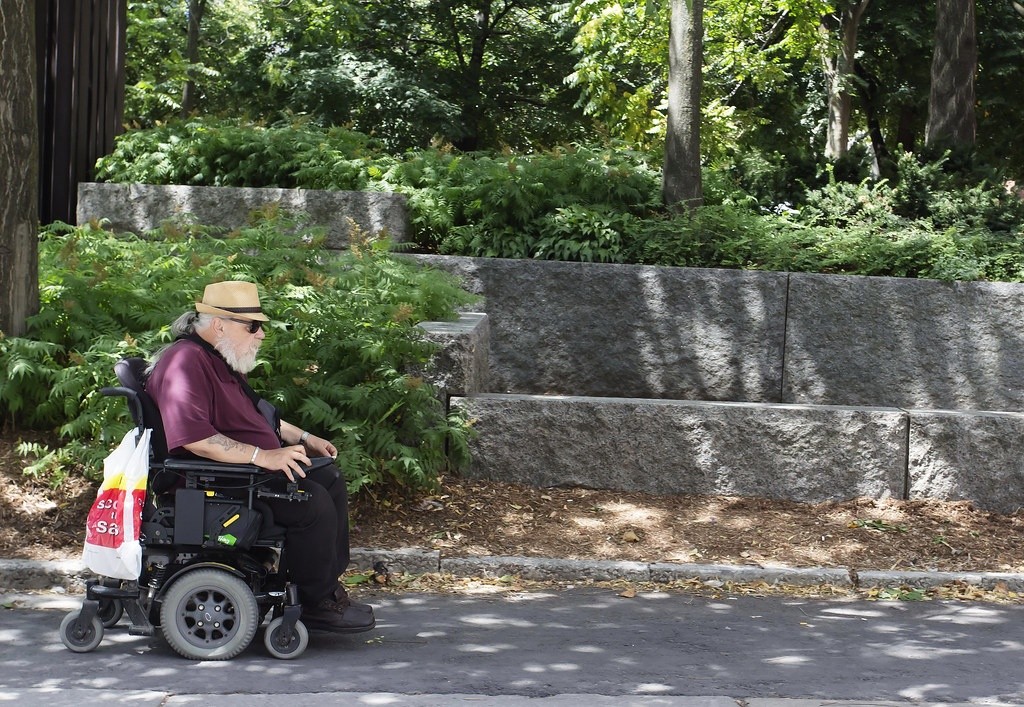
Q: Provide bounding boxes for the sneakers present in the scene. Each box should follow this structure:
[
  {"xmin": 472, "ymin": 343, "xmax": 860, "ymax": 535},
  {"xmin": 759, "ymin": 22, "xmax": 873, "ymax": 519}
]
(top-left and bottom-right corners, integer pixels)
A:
[{"xmin": 300, "ymin": 581, "xmax": 376, "ymax": 634}]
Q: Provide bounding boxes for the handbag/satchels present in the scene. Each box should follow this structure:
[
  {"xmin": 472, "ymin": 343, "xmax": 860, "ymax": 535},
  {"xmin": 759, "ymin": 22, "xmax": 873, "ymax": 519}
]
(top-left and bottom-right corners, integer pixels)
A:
[
  {"xmin": 82, "ymin": 427, "xmax": 153, "ymax": 582},
  {"xmin": 256, "ymin": 398, "xmax": 282, "ymax": 441}
]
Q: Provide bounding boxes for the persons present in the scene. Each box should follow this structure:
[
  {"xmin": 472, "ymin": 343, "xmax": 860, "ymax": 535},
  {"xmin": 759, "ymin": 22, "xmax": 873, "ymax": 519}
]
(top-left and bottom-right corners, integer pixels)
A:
[{"xmin": 144, "ymin": 281, "xmax": 376, "ymax": 633}]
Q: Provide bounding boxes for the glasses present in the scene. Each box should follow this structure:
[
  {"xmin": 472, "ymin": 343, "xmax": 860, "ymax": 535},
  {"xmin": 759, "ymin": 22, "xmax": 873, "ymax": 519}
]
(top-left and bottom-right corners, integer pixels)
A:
[{"xmin": 228, "ymin": 317, "xmax": 262, "ymax": 334}]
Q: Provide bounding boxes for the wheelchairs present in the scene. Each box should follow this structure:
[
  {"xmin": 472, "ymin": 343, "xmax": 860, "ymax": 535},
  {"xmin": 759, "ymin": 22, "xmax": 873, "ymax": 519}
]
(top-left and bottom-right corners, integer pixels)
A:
[{"xmin": 58, "ymin": 358, "xmax": 334, "ymax": 660}]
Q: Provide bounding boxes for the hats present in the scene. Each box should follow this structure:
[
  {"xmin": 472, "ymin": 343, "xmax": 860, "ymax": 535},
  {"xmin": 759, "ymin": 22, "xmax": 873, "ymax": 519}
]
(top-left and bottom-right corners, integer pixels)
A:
[{"xmin": 195, "ymin": 281, "xmax": 271, "ymax": 322}]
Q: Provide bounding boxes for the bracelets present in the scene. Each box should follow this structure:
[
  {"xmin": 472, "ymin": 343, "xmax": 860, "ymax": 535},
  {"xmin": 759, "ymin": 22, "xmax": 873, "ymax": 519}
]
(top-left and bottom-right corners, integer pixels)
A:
[
  {"xmin": 300, "ymin": 432, "xmax": 310, "ymax": 444},
  {"xmin": 250, "ymin": 447, "xmax": 259, "ymax": 464}
]
